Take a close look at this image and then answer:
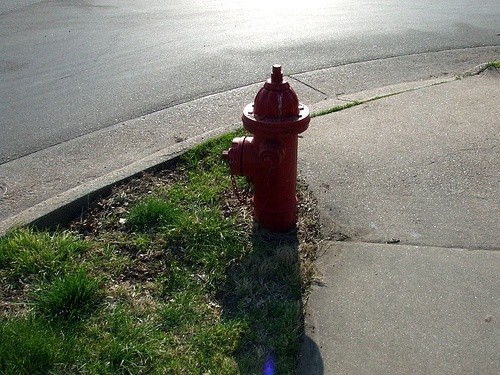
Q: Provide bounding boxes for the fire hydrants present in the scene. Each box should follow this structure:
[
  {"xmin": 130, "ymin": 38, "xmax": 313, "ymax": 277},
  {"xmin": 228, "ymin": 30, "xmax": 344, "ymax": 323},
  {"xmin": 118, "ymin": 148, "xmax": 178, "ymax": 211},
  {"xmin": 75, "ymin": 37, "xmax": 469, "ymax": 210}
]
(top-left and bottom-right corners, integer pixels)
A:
[{"xmin": 221, "ymin": 62, "xmax": 313, "ymax": 232}]
